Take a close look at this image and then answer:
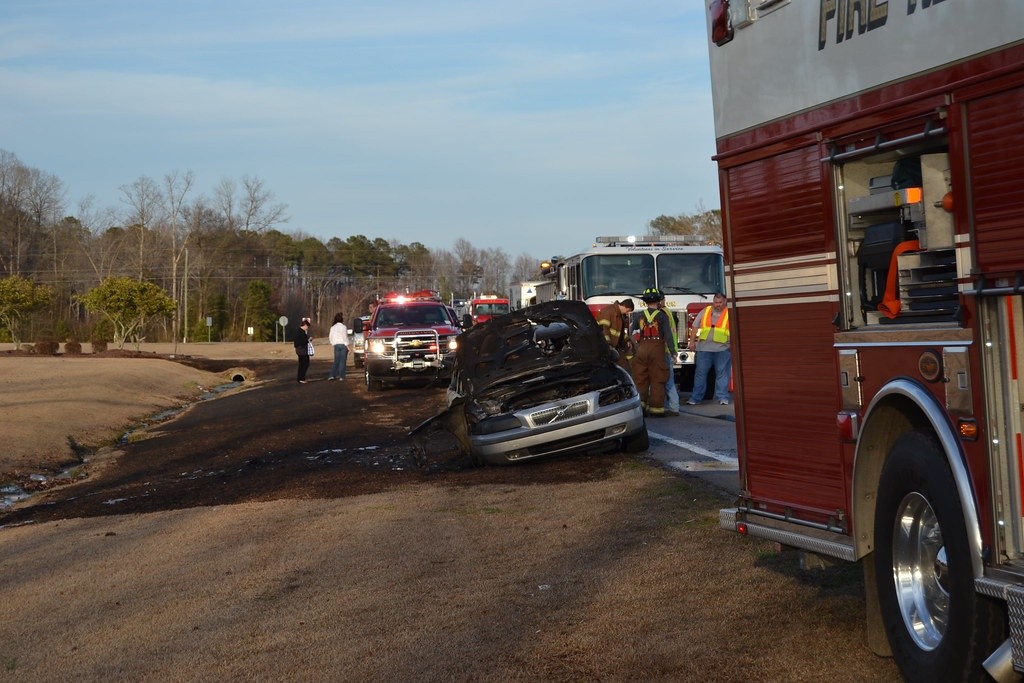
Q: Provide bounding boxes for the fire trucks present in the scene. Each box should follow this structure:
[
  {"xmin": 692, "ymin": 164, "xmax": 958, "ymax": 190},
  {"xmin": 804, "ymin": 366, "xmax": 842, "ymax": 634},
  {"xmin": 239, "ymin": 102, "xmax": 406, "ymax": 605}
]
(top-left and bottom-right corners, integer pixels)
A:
[
  {"xmin": 460, "ymin": 296, "xmax": 510, "ymax": 327},
  {"xmin": 534, "ymin": 236, "xmax": 728, "ymax": 400},
  {"xmin": 703, "ymin": 0, "xmax": 1024, "ymax": 683}
]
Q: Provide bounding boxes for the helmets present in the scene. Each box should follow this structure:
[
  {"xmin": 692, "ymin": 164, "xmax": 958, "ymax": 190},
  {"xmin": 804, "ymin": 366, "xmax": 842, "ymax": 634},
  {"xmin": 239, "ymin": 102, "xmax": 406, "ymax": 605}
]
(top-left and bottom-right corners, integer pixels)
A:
[{"xmin": 640, "ymin": 287, "xmax": 659, "ymax": 298}]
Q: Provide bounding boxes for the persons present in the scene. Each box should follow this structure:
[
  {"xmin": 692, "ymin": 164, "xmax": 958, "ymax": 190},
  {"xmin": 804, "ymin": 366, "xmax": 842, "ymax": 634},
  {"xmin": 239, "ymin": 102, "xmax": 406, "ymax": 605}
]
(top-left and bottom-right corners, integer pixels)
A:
[
  {"xmin": 294, "ymin": 321, "xmax": 313, "ymax": 384},
  {"xmin": 685, "ymin": 291, "xmax": 730, "ymax": 405},
  {"xmin": 596, "ymin": 287, "xmax": 680, "ymax": 417},
  {"xmin": 327, "ymin": 312, "xmax": 352, "ymax": 381},
  {"xmin": 369, "ymin": 299, "xmax": 378, "ymax": 313}
]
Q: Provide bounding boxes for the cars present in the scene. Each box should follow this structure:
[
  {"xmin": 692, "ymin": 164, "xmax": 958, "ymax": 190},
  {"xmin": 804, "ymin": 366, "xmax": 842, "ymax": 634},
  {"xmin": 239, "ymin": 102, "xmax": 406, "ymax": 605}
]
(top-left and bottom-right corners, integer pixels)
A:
[
  {"xmin": 347, "ymin": 315, "xmax": 371, "ymax": 370},
  {"xmin": 448, "ymin": 299, "xmax": 650, "ymax": 472}
]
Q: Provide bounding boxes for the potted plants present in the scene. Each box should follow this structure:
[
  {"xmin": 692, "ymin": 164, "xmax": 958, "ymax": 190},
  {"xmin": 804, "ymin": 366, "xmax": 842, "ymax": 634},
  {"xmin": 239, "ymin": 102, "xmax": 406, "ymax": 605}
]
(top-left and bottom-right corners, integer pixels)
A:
[{"xmin": 26, "ymin": 313, "xmax": 114, "ymax": 354}]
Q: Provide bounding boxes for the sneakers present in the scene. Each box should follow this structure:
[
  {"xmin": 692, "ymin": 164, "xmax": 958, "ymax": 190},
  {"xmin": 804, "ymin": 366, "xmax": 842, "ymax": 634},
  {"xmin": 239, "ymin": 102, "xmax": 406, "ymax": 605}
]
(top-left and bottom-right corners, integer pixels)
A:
[
  {"xmin": 720, "ymin": 400, "xmax": 728, "ymax": 405},
  {"xmin": 686, "ymin": 400, "xmax": 701, "ymax": 405}
]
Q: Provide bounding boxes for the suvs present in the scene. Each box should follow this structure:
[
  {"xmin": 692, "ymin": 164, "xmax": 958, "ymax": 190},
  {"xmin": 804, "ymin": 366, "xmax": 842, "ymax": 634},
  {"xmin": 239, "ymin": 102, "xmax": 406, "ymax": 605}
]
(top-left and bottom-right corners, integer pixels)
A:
[{"xmin": 352, "ymin": 291, "xmax": 474, "ymax": 393}]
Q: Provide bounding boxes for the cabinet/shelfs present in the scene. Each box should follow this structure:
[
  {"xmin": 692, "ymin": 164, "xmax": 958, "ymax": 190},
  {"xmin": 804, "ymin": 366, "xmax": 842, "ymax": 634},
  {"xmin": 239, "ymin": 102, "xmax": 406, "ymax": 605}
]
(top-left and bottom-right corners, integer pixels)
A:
[{"xmin": 897, "ymin": 248, "xmax": 956, "ymax": 311}]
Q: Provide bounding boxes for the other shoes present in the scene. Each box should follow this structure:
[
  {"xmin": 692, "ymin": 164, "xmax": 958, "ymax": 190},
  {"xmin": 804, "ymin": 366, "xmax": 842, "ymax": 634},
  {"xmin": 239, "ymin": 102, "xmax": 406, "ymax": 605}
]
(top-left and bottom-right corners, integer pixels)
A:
[
  {"xmin": 328, "ymin": 377, "xmax": 335, "ymax": 381},
  {"xmin": 642, "ymin": 407, "xmax": 646, "ymax": 414},
  {"xmin": 339, "ymin": 377, "xmax": 343, "ymax": 381},
  {"xmin": 297, "ymin": 380, "xmax": 306, "ymax": 383},
  {"xmin": 650, "ymin": 412, "xmax": 669, "ymax": 417},
  {"xmin": 664, "ymin": 410, "xmax": 679, "ymax": 416}
]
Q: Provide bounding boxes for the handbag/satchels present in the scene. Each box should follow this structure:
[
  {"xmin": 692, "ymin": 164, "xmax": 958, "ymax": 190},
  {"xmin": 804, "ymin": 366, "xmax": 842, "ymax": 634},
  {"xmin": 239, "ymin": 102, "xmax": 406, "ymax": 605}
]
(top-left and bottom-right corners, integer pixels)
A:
[{"xmin": 307, "ymin": 340, "xmax": 314, "ymax": 355}]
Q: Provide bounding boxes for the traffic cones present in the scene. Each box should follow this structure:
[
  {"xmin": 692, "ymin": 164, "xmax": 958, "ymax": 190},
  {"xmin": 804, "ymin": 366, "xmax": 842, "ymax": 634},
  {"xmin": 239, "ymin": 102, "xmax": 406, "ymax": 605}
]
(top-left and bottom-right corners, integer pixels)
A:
[{"xmin": 728, "ymin": 365, "xmax": 734, "ymax": 393}]
